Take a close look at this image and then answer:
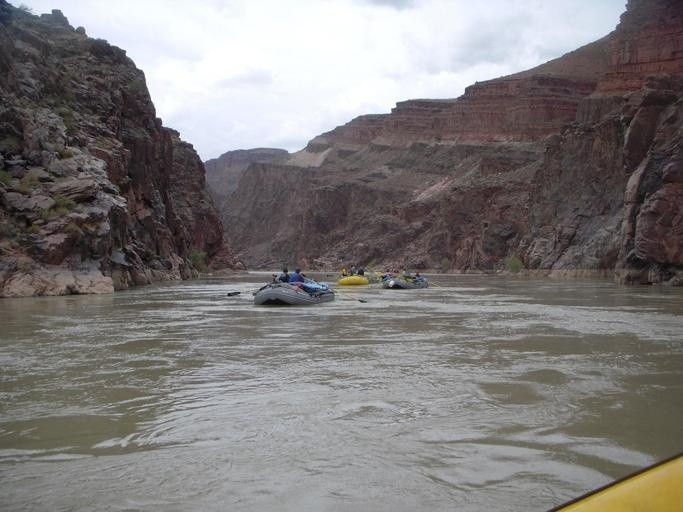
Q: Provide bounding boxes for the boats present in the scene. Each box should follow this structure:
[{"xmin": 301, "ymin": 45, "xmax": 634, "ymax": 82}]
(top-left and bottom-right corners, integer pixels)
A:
[
  {"xmin": 377, "ymin": 270, "xmax": 427, "ymax": 289},
  {"xmin": 253, "ymin": 275, "xmax": 334, "ymax": 305},
  {"xmin": 338, "ymin": 271, "xmax": 369, "ymax": 285}
]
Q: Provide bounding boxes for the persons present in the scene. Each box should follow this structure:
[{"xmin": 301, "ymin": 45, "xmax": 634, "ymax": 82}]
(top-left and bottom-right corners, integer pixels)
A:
[
  {"xmin": 278, "ymin": 269, "xmax": 289, "ymax": 282},
  {"xmin": 339, "ymin": 264, "xmax": 421, "ymax": 285},
  {"xmin": 288, "ymin": 269, "xmax": 303, "ymax": 283}
]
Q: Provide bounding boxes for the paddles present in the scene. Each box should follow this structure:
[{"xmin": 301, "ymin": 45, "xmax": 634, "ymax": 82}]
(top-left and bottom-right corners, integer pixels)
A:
[
  {"xmin": 303, "ymin": 275, "xmax": 367, "ymax": 304},
  {"xmin": 227, "ymin": 287, "xmax": 261, "ymax": 296},
  {"xmin": 326, "ymin": 275, "xmax": 342, "ymax": 277}
]
